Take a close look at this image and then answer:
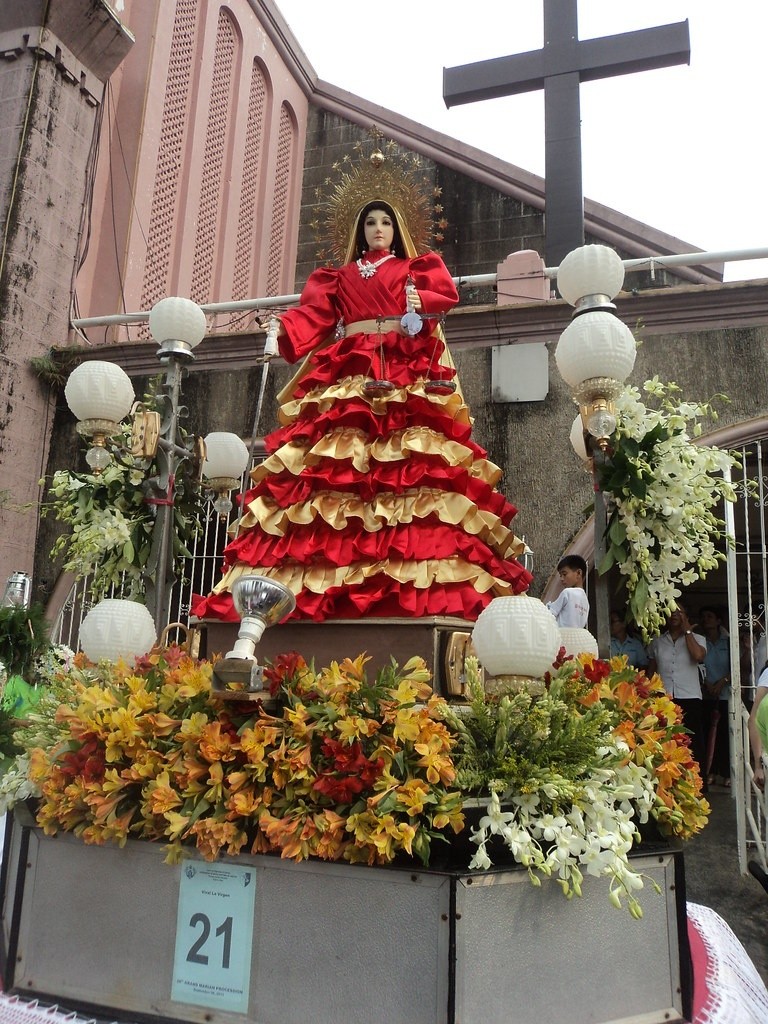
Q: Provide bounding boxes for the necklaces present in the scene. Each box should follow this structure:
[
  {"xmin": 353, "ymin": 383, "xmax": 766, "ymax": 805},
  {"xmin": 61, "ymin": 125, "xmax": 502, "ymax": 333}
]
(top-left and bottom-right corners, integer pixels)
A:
[{"xmin": 356, "ymin": 254, "xmax": 396, "ymax": 279}]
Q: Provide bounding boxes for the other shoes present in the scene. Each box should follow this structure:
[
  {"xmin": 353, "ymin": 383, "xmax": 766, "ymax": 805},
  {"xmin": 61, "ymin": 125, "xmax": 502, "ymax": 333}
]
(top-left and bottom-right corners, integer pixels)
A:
[
  {"xmin": 724, "ymin": 778, "xmax": 731, "ymax": 786},
  {"xmin": 707, "ymin": 774, "xmax": 714, "ymax": 784}
]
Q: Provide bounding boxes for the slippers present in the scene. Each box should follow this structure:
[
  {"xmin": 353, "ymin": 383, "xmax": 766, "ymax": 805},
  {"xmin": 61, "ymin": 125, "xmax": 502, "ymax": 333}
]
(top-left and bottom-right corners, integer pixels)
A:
[{"xmin": 748, "ymin": 861, "xmax": 768, "ymax": 895}]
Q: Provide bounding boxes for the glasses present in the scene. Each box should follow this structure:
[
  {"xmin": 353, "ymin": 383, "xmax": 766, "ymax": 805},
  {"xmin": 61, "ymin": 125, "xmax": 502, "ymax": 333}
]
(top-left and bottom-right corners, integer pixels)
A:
[{"xmin": 611, "ymin": 619, "xmax": 620, "ymax": 624}]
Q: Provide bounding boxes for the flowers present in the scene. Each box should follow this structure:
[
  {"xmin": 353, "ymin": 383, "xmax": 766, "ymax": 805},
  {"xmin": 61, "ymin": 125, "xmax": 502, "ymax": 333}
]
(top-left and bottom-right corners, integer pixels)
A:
[{"xmin": 0, "ymin": 313, "xmax": 758, "ymax": 923}]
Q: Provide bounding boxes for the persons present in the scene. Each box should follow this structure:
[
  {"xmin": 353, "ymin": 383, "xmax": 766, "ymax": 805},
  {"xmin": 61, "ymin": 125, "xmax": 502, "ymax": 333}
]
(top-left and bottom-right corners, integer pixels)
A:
[
  {"xmin": 546, "ymin": 555, "xmax": 590, "ymax": 628},
  {"xmin": 189, "ymin": 200, "xmax": 534, "ymax": 624},
  {"xmin": 609, "ymin": 601, "xmax": 768, "ymax": 793}
]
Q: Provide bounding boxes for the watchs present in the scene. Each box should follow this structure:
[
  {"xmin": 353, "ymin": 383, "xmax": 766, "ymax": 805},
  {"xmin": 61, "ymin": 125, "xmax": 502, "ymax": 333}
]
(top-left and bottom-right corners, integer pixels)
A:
[{"xmin": 685, "ymin": 629, "xmax": 692, "ymax": 636}]
